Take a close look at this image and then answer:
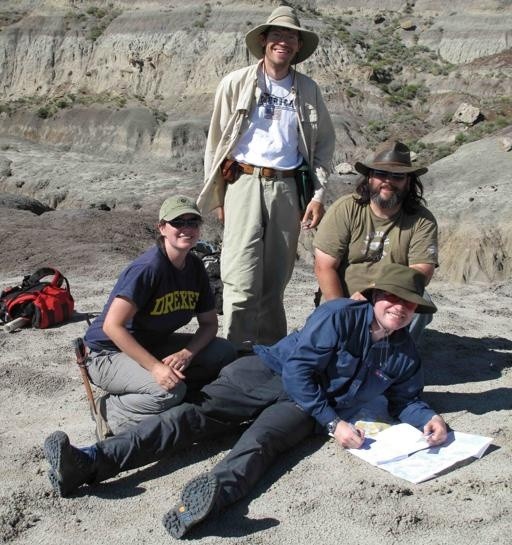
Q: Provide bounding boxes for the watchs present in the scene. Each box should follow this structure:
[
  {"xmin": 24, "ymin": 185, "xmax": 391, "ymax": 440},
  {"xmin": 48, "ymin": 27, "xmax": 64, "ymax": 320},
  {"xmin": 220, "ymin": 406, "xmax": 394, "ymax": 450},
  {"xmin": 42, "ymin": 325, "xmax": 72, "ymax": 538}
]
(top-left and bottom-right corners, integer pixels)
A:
[{"xmin": 326, "ymin": 417, "xmax": 340, "ymax": 434}]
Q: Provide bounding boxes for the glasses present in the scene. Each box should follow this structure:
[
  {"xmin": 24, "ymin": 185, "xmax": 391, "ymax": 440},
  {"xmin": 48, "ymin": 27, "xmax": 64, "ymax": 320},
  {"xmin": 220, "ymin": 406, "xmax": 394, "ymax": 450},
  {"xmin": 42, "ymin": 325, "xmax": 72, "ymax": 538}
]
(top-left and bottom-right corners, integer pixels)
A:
[{"xmin": 164, "ymin": 217, "xmax": 201, "ymax": 229}]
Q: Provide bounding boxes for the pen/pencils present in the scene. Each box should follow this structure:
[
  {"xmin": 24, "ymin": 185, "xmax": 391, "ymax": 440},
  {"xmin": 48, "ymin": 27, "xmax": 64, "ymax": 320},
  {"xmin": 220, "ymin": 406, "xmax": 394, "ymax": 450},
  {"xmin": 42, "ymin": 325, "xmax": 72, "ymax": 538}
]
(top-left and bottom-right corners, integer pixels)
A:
[{"xmin": 349, "ymin": 423, "xmax": 360, "ymax": 437}]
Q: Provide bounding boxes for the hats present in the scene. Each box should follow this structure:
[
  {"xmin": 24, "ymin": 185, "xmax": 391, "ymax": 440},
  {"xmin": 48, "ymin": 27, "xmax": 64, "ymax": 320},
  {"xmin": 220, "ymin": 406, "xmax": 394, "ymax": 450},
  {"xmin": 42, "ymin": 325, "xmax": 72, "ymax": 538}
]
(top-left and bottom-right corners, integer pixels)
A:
[
  {"xmin": 361, "ymin": 263, "xmax": 437, "ymax": 313},
  {"xmin": 159, "ymin": 195, "xmax": 201, "ymax": 222},
  {"xmin": 355, "ymin": 140, "xmax": 427, "ymax": 177},
  {"xmin": 245, "ymin": 6, "xmax": 318, "ymax": 65}
]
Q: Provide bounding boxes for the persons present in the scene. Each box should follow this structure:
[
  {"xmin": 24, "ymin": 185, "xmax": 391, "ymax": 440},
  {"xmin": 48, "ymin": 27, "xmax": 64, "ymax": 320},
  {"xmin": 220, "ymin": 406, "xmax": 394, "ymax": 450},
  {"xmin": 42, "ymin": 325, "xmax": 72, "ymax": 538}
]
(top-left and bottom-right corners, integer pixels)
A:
[
  {"xmin": 196, "ymin": 5, "xmax": 336, "ymax": 346},
  {"xmin": 80, "ymin": 194, "xmax": 240, "ymax": 434},
  {"xmin": 42, "ymin": 263, "xmax": 447, "ymax": 539},
  {"xmin": 312, "ymin": 141, "xmax": 443, "ymax": 306}
]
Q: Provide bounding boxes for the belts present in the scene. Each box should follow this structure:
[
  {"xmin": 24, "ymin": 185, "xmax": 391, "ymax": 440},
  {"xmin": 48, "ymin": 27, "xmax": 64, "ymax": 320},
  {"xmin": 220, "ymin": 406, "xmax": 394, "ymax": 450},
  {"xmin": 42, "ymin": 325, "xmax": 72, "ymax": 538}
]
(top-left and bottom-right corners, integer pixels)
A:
[{"xmin": 237, "ymin": 163, "xmax": 296, "ymax": 177}]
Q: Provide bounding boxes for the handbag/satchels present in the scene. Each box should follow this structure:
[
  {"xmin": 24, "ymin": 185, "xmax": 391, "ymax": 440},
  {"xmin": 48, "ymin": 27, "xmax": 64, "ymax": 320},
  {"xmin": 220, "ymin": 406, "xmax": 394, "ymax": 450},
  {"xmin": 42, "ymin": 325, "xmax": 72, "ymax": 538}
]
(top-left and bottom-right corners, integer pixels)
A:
[{"xmin": 0, "ymin": 267, "xmax": 73, "ymax": 329}]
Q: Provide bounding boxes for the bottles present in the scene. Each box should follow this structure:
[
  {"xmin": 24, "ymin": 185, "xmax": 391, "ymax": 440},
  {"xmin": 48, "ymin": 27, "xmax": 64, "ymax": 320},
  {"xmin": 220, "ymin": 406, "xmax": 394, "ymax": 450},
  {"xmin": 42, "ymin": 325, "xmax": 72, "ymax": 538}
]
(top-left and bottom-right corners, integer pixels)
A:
[{"xmin": 296, "ymin": 170, "xmax": 314, "ymax": 214}]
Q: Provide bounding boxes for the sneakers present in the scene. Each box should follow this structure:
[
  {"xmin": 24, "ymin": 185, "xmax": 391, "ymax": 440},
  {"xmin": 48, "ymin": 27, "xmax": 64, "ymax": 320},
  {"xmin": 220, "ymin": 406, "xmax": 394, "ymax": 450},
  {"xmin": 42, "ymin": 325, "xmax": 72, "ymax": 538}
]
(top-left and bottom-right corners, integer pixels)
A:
[
  {"xmin": 161, "ymin": 472, "xmax": 222, "ymax": 539},
  {"xmin": 90, "ymin": 394, "xmax": 113, "ymax": 443},
  {"xmin": 44, "ymin": 431, "xmax": 93, "ymax": 497}
]
